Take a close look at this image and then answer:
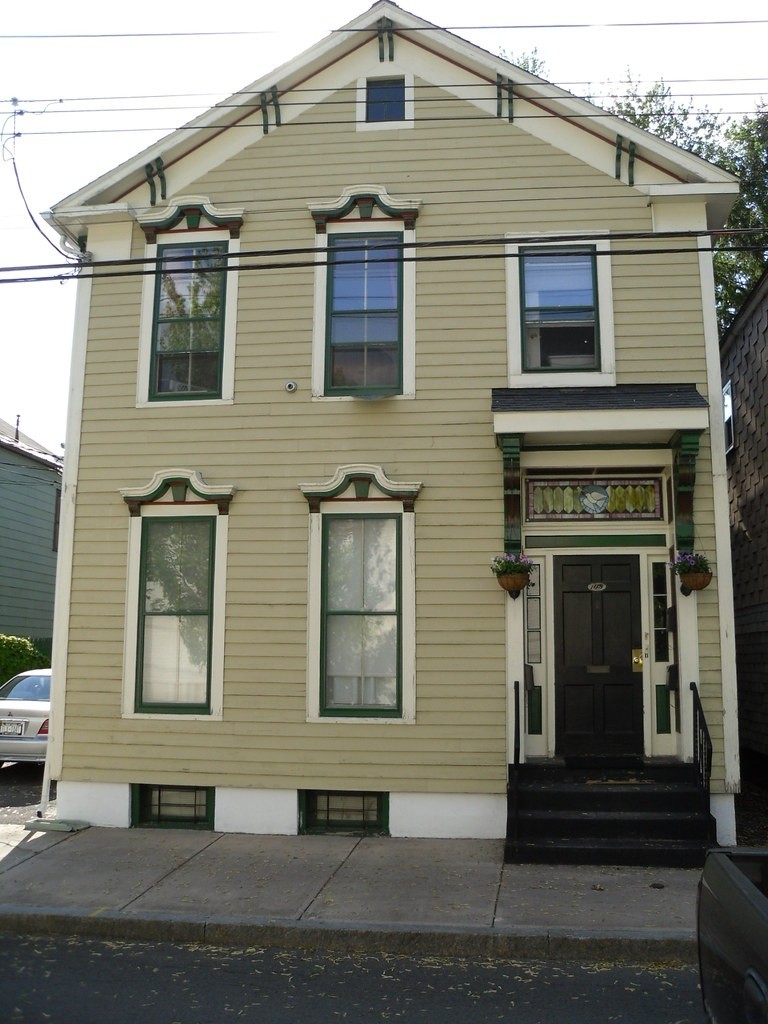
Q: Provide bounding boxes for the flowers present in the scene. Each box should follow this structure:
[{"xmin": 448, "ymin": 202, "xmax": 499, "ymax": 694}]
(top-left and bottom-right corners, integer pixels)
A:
[
  {"xmin": 490, "ymin": 553, "xmax": 538, "ymax": 574},
  {"xmin": 667, "ymin": 552, "xmax": 712, "ymax": 575}
]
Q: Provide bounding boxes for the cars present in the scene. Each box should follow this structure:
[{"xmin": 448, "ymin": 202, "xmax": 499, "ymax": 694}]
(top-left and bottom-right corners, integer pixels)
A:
[{"xmin": 0, "ymin": 668, "xmax": 52, "ymax": 767}]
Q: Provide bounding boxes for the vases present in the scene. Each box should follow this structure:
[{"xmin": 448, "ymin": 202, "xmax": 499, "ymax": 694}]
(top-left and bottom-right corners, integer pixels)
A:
[
  {"xmin": 496, "ymin": 573, "xmax": 530, "ymax": 591},
  {"xmin": 680, "ymin": 573, "xmax": 713, "ymax": 590}
]
